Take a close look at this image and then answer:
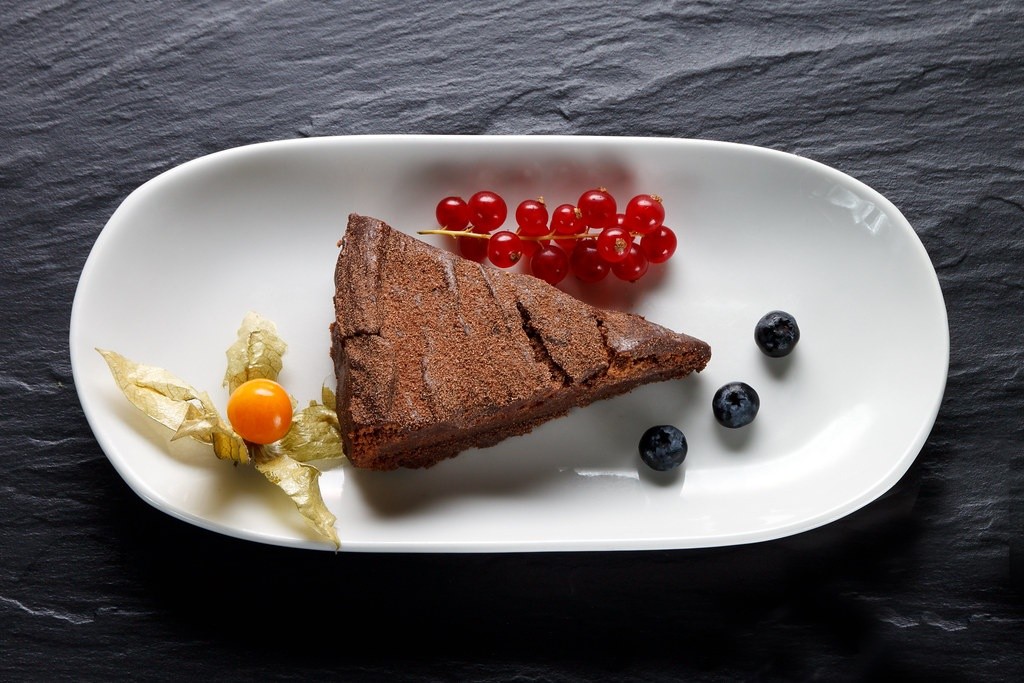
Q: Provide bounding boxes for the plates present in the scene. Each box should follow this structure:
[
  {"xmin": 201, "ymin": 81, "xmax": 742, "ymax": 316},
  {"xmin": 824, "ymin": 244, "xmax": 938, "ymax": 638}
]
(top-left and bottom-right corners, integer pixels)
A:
[{"xmin": 68, "ymin": 135, "xmax": 951, "ymax": 553}]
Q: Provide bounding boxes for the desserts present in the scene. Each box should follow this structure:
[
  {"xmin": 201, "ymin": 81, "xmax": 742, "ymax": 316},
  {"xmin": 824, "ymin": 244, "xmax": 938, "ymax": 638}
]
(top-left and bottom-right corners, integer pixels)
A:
[{"xmin": 328, "ymin": 213, "xmax": 712, "ymax": 471}]
